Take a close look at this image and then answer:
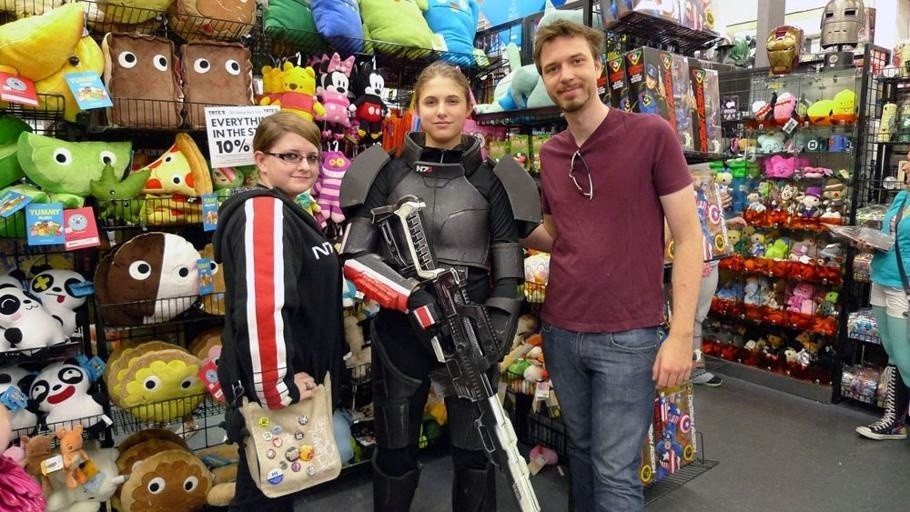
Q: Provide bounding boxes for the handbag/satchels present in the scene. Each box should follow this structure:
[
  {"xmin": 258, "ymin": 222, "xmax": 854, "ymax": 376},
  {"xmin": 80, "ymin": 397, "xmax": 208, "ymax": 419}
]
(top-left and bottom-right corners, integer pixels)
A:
[{"xmin": 239, "ymin": 371, "xmax": 343, "ymax": 500}]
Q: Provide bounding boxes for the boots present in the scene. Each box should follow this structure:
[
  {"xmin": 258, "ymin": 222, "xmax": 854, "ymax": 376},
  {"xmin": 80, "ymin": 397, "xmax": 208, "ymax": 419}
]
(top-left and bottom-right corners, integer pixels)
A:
[{"xmin": 855, "ymin": 364, "xmax": 908, "ymax": 441}]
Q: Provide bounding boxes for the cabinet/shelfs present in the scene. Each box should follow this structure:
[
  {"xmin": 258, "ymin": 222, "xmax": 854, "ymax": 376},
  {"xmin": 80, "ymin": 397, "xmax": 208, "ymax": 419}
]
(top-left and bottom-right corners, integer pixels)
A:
[
  {"xmin": 828, "ymin": 43, "xmax": 910, "ymax": 417},
  {"xmin": 0, "ymin": 0, "xmax": 510, "ymax": 512},
  {"xmin": 473, "ymin": 0, "xmax": 722, "ymax": 490},
  {"xmin": 701, "ymin": 43, "xmax": 892, "ymax": 406}
]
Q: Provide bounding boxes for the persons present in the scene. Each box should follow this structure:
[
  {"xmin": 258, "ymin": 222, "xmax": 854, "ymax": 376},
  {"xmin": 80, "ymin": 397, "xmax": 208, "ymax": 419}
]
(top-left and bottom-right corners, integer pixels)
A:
[
  {"xmin": 528, "ymin": 16, "xmax": 706, "ymax": 512},
  {"xmin": 854, "ymin": 190, "xmax": 909, "ymax": 443}
]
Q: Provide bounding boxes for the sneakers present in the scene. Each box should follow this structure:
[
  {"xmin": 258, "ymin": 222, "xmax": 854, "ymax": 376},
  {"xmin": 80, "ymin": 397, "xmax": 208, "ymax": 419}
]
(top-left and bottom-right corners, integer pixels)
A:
[{"xmin": 691, "ymin": 366, "xmax": 723, "ymax": 387}]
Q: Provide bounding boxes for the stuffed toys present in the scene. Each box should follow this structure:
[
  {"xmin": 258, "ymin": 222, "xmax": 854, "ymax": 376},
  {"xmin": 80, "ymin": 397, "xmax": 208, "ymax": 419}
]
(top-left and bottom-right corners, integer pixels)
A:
[
  {"xmin": 699, "ymin": 89, "xmax": 857, "ymax": 378},
  {"xmin": 0, "ymin": 0, "xmax": 604, "ymax": 512},
  {"xmin": 605, "ymin": 0, "xmax": 909, "ymax": 486}
]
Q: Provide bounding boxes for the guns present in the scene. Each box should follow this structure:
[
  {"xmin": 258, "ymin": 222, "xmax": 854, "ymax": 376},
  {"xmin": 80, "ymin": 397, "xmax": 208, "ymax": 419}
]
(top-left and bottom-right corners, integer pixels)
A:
[{"xmin": 370, "ymin": 193, "xmax": 541, "ymax": 511}]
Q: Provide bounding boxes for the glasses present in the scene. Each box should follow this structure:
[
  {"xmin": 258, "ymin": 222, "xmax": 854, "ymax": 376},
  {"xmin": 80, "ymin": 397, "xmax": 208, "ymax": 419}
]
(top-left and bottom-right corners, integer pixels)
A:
[
  {"xmin": 567, "ymin": 151, "xmax": 594, "ymax": 200},
  {"xmin": 264, "ymin": 151, "xmax": 319, "ymax": 166}
]
